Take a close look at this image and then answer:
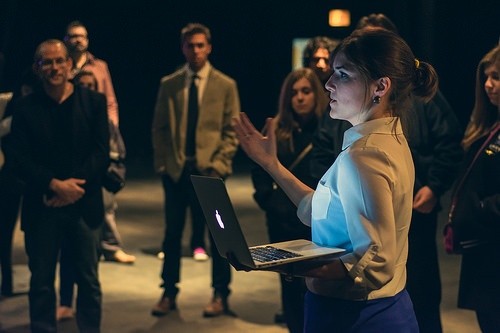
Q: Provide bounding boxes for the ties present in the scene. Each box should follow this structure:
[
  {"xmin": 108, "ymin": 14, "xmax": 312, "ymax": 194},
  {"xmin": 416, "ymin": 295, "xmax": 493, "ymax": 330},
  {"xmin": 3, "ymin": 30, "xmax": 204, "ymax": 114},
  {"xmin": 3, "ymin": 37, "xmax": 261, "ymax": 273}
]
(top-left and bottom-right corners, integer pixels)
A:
[{"xmin": 185, "ymin": 75, "xmax": 199, "ymax": 173}]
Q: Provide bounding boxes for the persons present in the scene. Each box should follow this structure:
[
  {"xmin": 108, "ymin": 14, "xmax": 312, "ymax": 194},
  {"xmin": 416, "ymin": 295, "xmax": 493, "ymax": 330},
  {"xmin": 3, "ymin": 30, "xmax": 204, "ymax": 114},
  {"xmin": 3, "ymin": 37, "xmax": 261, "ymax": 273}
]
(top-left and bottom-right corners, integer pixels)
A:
[{"xmin": 0, "ymin": 14, "xmax": 500, "ymax": 333}]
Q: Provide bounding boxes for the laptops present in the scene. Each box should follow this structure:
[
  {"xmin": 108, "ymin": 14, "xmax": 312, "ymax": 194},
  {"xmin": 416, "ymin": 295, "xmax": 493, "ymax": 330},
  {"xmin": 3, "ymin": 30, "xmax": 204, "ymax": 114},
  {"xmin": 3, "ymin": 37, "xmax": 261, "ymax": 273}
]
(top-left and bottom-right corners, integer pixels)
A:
[{"xmin": 190, "ymin": 175, "xmax": 347, "ymax": 271}]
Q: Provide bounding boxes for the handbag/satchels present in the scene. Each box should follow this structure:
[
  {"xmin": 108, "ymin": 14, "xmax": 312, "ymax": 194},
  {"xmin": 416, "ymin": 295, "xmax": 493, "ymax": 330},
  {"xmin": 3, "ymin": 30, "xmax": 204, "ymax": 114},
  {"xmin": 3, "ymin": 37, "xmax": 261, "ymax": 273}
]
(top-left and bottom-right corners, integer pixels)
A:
[
  {"xmin": 443, "ymin": 214, "xmax": 459, "ymax": 255},
  {"xmin": 253, "ymin": 188, "xmax": 277, "ymax": 211}
]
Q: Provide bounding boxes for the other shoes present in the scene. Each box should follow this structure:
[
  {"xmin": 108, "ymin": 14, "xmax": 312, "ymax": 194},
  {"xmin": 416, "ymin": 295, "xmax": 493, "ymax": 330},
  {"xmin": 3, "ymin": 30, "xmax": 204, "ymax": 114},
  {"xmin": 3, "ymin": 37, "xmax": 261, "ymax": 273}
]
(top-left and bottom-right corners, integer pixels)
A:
[
  {"xmin": 152, "ymin": 295, "xmax": 176, "ymax": 316},
  {"xmin": 194, "ymin": 247, "xmax": 208, "ymax": 261},
  {"xmin": 203, "ymin": 293, "xmax": 229, "ymax": 317},
  {"xmin": 56, "ymin": 307, "xmax": 74, "ymax": 320},
  {"xmin": 102, "ymin": 250, "xmax": 136, "ymax": 263}
]
